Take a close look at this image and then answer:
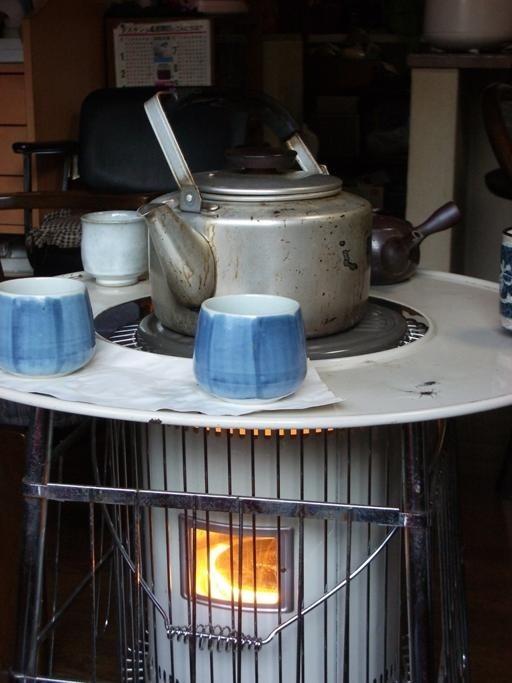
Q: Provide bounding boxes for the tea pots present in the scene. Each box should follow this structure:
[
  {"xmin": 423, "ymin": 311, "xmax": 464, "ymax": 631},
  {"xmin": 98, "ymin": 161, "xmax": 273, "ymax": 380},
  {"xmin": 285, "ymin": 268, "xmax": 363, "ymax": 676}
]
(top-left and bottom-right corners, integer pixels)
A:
[
  {"xmin": 136, "ymin": 84, "xmax": 372, "ymax": 342},
  {"xmin": 370, "ymin": 200, "xmax": 466, "ymax": 285}
]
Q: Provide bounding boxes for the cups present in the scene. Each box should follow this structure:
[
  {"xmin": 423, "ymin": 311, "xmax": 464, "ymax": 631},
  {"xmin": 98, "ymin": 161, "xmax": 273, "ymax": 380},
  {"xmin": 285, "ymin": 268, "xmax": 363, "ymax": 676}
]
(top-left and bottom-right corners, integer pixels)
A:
[
  {"xmin": 497, "ymin": 227, "xmax": 511, "ymax": 331},
  {"xmin": 192, "ymin": 291, "xmax": 312, "ymax": 403},
  {"xmin": 79, "ymin": 208, "xmax": 150, "ymax": 287},
  {"xmin": 0, "ymin": 273, "xmax": 98, "ymax": 376}
]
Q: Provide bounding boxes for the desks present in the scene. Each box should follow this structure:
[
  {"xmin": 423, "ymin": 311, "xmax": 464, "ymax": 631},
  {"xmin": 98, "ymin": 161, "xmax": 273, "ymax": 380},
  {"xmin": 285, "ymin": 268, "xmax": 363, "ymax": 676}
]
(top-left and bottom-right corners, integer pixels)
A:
[{"xmin": 0, "ymin": 267, "xmax": 512, "ymax": 683}]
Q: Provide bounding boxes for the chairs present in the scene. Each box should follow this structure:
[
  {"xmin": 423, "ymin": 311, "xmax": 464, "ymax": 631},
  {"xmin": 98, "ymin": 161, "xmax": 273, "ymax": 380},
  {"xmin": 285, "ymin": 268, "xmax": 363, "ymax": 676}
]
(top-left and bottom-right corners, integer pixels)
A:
[
  {"xmin": 12, "ymin": 139, "xmax": 110, "ymax": 276},
  {"xmin": 0, "ymin": 86, "xmax": 232, "ymax": 272}
]
